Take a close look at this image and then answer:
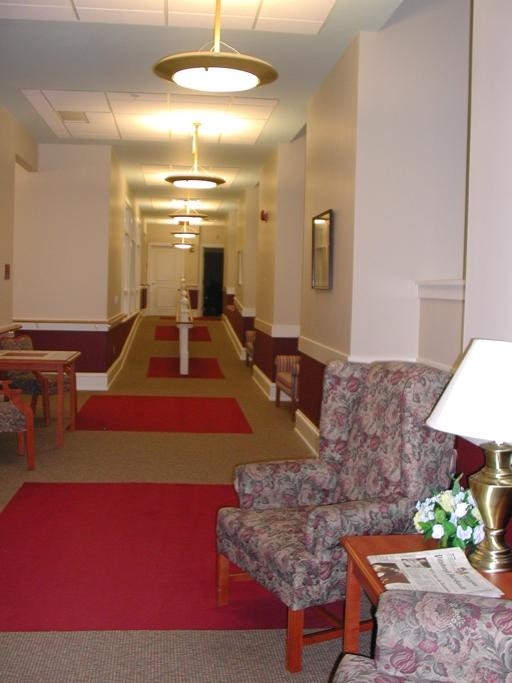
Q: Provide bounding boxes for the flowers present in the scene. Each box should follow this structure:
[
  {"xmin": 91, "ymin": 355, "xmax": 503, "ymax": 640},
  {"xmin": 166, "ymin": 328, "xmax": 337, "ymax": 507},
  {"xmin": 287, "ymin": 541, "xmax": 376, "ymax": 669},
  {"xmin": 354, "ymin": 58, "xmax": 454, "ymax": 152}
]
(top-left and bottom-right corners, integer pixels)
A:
[{"xmin": 413, "ymin": 472, "xmax": 486, "ymax": 551}]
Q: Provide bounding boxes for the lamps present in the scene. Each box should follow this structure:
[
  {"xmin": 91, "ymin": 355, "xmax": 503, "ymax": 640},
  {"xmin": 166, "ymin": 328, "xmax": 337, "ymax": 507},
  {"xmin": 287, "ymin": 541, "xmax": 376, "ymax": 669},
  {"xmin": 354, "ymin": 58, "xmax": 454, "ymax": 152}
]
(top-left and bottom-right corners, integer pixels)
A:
[
  {"xmin": 165, "ymin": 123, "xmax": 227, "ymax": 251},
  {"xmin": 152, "ymin": 0, "xmax": 280, "ymax": 94},
  {"xmin": 426, "ymin": 338, "xmax": 511, "ymax": 574}
]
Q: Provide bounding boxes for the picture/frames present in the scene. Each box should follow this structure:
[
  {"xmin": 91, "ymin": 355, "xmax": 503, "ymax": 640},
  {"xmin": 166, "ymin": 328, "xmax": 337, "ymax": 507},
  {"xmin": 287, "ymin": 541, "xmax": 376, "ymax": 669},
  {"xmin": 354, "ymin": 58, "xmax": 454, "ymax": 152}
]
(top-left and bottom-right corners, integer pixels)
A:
[{"xmin": 311, "ymin": 209, "xmax": 334, "ymax": 290}]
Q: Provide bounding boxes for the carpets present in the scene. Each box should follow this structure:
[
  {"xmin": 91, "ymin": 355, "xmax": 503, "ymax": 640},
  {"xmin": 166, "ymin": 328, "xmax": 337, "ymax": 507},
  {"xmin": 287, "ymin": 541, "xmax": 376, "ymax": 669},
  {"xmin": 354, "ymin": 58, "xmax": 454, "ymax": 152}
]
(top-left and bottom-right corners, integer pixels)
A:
[
  {"xmin": 67, "ymin": 394, "xmax": 255, "ymax": 433},
  {"xmin": 1, "ymin": 481, "xmax": 347, "ymax": 630},
  {"xmin": 146, "ymin": 314, "xmax": 227, "ymax": 379}
]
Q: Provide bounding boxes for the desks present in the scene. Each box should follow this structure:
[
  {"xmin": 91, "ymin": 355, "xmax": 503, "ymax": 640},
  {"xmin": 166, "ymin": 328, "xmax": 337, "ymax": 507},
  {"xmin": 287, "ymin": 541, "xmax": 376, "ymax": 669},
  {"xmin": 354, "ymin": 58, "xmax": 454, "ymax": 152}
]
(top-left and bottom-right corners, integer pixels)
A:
[{"xmin": 0, "ymin": 349, "xmax": 82, "ymax": 449}]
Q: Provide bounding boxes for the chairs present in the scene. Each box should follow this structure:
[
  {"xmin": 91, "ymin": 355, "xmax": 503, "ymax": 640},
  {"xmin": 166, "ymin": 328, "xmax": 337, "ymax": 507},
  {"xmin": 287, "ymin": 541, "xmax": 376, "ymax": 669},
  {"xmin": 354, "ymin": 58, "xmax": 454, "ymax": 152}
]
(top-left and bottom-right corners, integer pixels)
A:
[
  {"xmin": 216, "ymin": 359, "xmax": 457, "ymax": 673},
  {"xmin": 0, "ymin": 335, "xmax": 69, "ymax": 416}
]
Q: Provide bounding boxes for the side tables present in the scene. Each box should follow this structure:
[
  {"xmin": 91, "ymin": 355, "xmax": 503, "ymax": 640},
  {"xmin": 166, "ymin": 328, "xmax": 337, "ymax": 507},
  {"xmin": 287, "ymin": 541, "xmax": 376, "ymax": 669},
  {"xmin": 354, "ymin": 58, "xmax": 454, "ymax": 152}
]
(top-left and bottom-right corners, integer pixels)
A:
[{"xmin": 339, "ymin": 534, "xmax": 511, "ymax": 658}]
